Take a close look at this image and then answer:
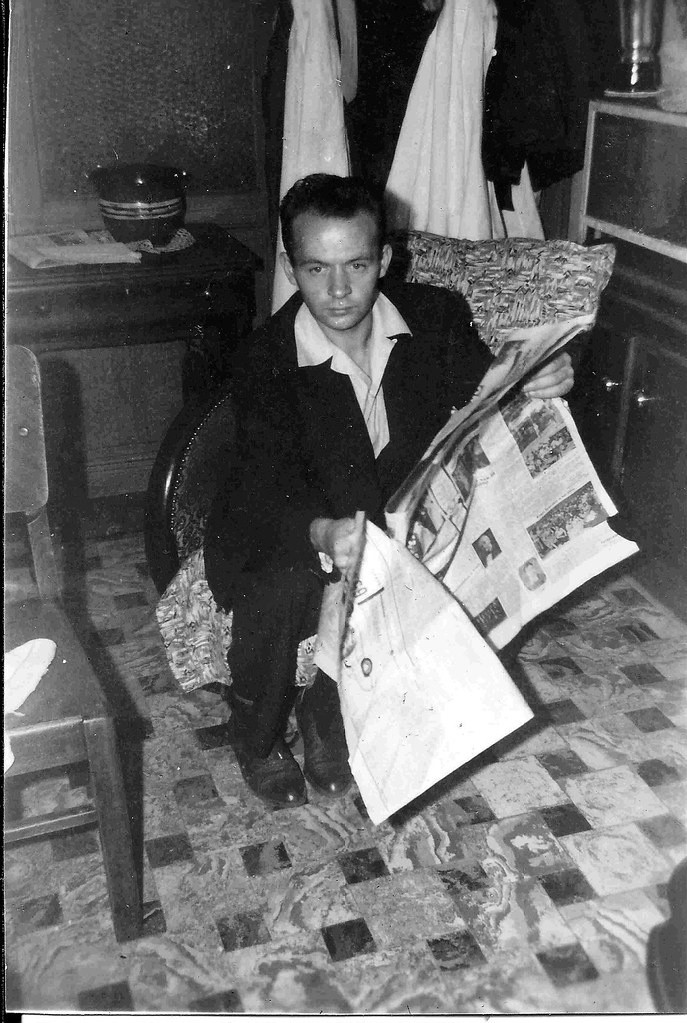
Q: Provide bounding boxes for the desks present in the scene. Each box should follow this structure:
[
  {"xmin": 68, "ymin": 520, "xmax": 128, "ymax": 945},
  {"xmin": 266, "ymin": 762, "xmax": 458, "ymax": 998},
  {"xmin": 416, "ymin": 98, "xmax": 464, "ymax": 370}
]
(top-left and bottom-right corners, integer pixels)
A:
[{"xmin": 6, "ymin": 219, "xmax": 266, "ymax": 404}]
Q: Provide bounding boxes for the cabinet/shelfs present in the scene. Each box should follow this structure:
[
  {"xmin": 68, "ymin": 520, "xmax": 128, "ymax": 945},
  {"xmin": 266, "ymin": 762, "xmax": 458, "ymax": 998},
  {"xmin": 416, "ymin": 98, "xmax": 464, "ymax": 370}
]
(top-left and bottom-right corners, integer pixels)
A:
[{"xmin": 565, "ymin": 234, "xmax": 687, "ymax": 624}]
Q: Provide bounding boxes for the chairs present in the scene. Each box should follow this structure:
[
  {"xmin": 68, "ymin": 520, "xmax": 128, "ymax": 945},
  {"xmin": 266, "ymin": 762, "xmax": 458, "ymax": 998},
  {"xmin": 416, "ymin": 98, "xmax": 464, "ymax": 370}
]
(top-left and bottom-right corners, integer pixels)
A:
[{"xmin": 4, "ymin": 343, "xmax": 142, "ymax": 947}]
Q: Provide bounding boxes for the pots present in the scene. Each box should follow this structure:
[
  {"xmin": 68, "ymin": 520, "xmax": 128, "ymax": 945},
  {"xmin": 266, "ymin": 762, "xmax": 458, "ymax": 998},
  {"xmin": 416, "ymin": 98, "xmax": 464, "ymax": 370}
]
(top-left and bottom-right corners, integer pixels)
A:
[{"xmin": 88, "ymin": 162, "xmax": 189, "ymax": 248}]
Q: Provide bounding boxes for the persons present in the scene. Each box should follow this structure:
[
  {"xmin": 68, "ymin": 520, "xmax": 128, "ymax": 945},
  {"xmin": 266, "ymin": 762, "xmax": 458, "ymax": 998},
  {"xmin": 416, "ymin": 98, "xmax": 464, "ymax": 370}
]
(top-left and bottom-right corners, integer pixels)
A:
[{"xmin": 201, "ymin": 172, "xmax": 576, "ymax": 807}]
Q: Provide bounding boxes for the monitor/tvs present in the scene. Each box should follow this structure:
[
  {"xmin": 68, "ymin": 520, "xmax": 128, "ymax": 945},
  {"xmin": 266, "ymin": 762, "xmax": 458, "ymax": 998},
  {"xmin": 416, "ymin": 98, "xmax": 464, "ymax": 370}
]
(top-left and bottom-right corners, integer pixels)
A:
[{"xmin": 583, "ymin": 101, "xmax": 687, "ymax": 266}]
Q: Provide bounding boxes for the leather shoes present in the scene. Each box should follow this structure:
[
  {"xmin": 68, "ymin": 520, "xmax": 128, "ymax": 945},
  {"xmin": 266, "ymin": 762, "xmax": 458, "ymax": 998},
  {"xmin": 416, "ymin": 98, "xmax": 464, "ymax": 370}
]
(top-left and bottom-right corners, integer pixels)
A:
[
  {"xmin": 296, "ymin": 700, "xmax": 352, "ymax": 799},
  {"xmin": 227, "ymin": 717, "xmax": 307, "ymax": 807}
]
